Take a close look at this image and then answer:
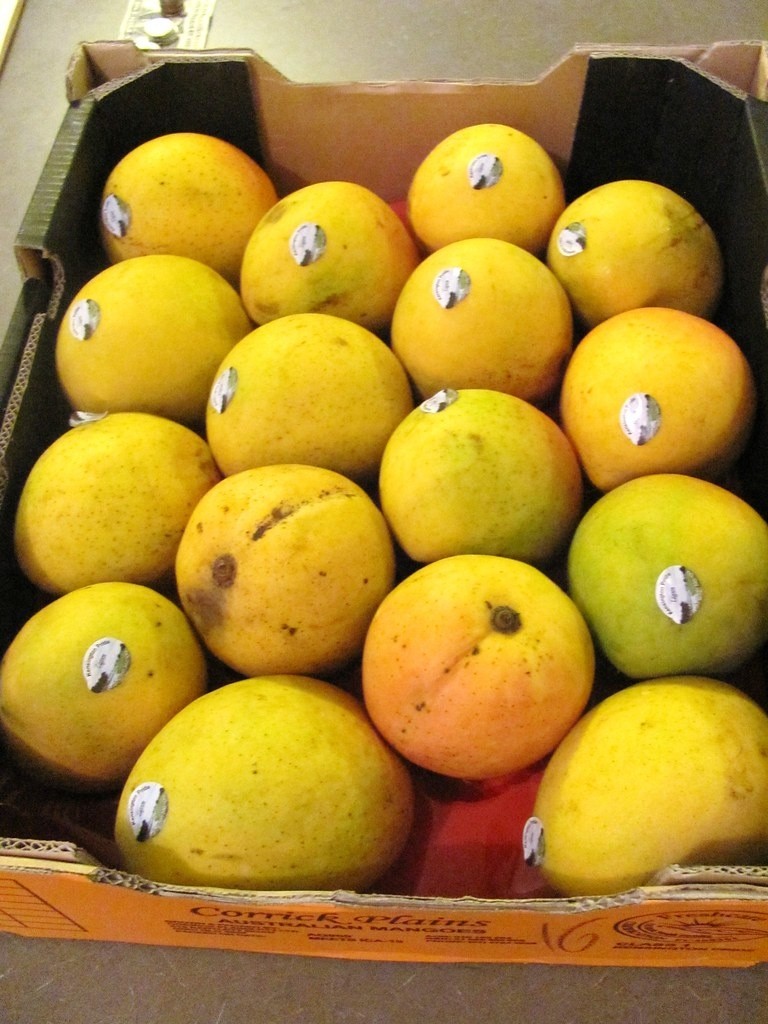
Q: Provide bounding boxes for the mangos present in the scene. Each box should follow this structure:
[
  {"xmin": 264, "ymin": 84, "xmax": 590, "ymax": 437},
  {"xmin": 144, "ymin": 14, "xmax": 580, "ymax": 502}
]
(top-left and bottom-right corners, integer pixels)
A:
[{"xmin": 0, "ymin": 124, "xmax": 768, "ymax": 894}]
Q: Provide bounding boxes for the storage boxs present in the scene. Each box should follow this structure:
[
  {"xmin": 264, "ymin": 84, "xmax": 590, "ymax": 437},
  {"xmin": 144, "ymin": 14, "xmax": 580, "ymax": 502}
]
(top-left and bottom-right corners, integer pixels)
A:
[{"xmin": 1, "ymin": 40, "xmax": 768, "ymax": 968}]
